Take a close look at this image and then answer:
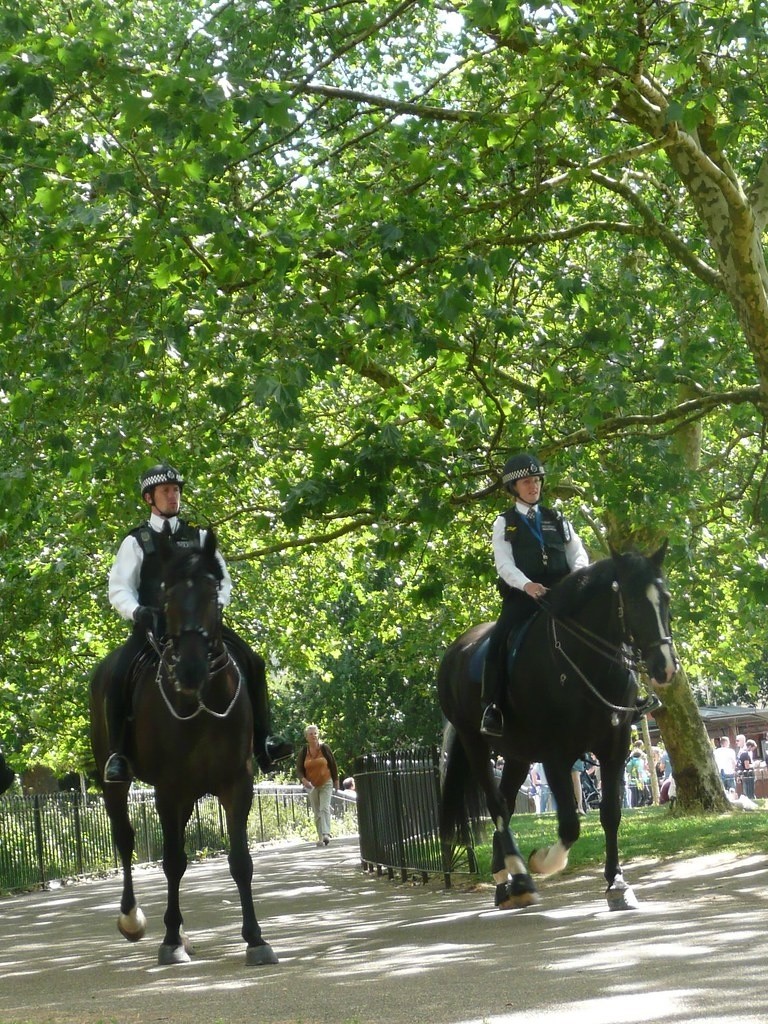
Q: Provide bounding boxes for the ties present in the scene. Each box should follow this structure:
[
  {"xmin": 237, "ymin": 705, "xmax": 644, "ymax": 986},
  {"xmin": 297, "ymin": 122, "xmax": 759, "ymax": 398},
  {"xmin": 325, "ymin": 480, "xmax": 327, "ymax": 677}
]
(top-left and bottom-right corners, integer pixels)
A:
[
  {"xmin": 527, "ymin": 507, "xmax": 537, "ymax": 522},
  {"xmin": 163, "ymin": 520, "xmax": 171, "ymax": 536}
]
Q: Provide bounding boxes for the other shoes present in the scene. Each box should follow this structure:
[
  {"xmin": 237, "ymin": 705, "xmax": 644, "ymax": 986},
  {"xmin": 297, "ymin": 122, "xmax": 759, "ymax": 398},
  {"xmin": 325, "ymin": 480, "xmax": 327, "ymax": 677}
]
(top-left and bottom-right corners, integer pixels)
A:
[
  {"xmin": 323, "ymin": 835, "xmax": 330, "ymax": 846},
  {"xmin": 317, "ymin": 841, "xmax": 323, "ymax": 846}
]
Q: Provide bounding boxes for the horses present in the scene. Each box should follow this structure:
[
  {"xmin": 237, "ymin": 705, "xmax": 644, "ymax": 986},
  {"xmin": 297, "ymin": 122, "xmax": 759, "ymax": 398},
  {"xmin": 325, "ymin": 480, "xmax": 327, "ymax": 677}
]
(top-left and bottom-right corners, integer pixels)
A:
[
  {"xmin": 438, "ymin": 537, "xmax": 678, "ymax": 911},
  {"xmin": 90, "ymin": 520, "xmax": 278, "ymax": 965}
]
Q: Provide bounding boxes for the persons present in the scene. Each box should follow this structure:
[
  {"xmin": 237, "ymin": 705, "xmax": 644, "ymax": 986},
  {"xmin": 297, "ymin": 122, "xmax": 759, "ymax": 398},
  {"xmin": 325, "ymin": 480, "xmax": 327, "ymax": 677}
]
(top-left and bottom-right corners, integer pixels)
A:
[
  {"xmin": 103, "ymin": 466, "xmax": 297, "ymax": 785},
  {"xmin": 713, "ymin": 732, "xmax": 768, "ymax": 799},
  {"xmin": 482, "ymin": 452, "xmax": 663, "ymax": 737},
  {"xmin": 490, "ymin": 737, "xmax": 678, "ymax": 813},
  {"xmin": 342, "ymin": 777, "xmax": 357, "ymax": 813},
  {"xmin": 297, "ymin": 725, "xmax": 340, "ymax": 845}
]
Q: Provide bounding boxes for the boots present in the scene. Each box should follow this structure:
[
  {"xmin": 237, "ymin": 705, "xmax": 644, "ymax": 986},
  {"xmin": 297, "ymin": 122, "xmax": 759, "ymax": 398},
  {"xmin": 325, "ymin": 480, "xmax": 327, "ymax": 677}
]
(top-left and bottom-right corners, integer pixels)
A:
[
  {"xmin": 633, "ymin": 696, "xmax": 652, "ymax": 719},
  {"xmin": 102, "ymin": 679, "xmax": 132, "ymax": 781},
  {"xmin": 481, "ymin": 661, "xmax": 506, "ymax": 727},
  {"xmin": 248, "ymin": 679, "xmax": 296, "ymax": 773}
]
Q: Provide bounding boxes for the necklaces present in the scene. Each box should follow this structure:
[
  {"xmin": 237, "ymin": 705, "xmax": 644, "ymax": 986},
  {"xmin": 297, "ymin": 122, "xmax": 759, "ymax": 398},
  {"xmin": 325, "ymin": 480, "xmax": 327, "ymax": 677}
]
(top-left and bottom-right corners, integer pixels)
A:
[
  {"xmin": 521, "ymin": 511, "xmax": 545, "ymax": 545},
  {"xmin": 308, "ymin": 746, "xmax": 320, "ymax": 759}
]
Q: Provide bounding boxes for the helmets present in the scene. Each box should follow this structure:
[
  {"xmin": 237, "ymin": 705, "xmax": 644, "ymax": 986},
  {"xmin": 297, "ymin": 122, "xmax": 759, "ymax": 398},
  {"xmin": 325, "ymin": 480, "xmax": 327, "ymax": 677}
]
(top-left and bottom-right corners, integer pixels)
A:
[
  {"xmin": 141, "ymin": 465, "xmax": 185, "ymax": 498},
  {"xmin": 502, "ymin": 455, "xmax": 545, "ymax": 487}
]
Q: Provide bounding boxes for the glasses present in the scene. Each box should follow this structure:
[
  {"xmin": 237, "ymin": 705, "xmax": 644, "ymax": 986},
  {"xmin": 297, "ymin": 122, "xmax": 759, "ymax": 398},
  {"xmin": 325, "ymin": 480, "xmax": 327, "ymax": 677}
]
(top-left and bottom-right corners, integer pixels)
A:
[{"xmin": 736, "ymin": 740, "xmax": 739, "ymax": 742}]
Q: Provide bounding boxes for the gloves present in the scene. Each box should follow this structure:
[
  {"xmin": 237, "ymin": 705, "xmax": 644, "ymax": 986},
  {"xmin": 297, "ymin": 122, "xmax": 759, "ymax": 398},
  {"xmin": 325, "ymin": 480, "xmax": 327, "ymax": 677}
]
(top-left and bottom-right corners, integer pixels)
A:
[{"xmin": 133, "ymin": 606, "xmax": 160, "ymax": 624}]
